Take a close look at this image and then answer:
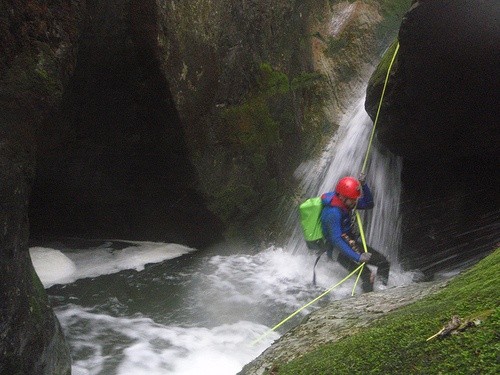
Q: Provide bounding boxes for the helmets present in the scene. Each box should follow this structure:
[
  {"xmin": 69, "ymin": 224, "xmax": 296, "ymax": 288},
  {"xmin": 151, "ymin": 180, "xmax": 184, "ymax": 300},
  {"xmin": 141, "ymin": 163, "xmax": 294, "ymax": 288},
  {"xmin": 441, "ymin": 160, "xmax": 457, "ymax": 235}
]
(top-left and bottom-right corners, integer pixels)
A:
[{"xmin": 335, "ymin": 176, "xmax": 361, "ymax": 200}]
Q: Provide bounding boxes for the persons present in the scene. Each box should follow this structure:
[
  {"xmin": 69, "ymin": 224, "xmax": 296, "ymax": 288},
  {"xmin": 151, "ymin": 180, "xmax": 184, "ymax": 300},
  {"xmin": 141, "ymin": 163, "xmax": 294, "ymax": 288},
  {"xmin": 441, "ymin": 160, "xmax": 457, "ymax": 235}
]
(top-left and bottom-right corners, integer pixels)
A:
[{"xmin": 321, "ymin": 171, "xmax": 392, "ymax": 293}]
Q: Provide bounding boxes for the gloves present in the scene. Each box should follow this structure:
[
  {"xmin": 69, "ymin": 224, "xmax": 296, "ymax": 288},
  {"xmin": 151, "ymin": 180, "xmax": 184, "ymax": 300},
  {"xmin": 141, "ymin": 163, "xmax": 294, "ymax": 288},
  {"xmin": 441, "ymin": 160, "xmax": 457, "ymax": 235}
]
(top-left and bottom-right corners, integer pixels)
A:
[
  {"xmin": 359, "ymin": 252, "xmax": 372, "ymax": 262},
  {"xmin": 358, "ymin": 172, "xmax": 367, "ymax": 184}
]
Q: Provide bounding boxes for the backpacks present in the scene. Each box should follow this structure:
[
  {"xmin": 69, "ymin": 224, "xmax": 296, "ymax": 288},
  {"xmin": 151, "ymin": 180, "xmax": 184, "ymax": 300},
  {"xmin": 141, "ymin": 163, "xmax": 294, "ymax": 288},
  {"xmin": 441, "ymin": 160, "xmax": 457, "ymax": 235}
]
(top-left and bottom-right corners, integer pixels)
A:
[{"xmin": 299, "ymin": 196, "xmax": 330, "ymax": 250}]
